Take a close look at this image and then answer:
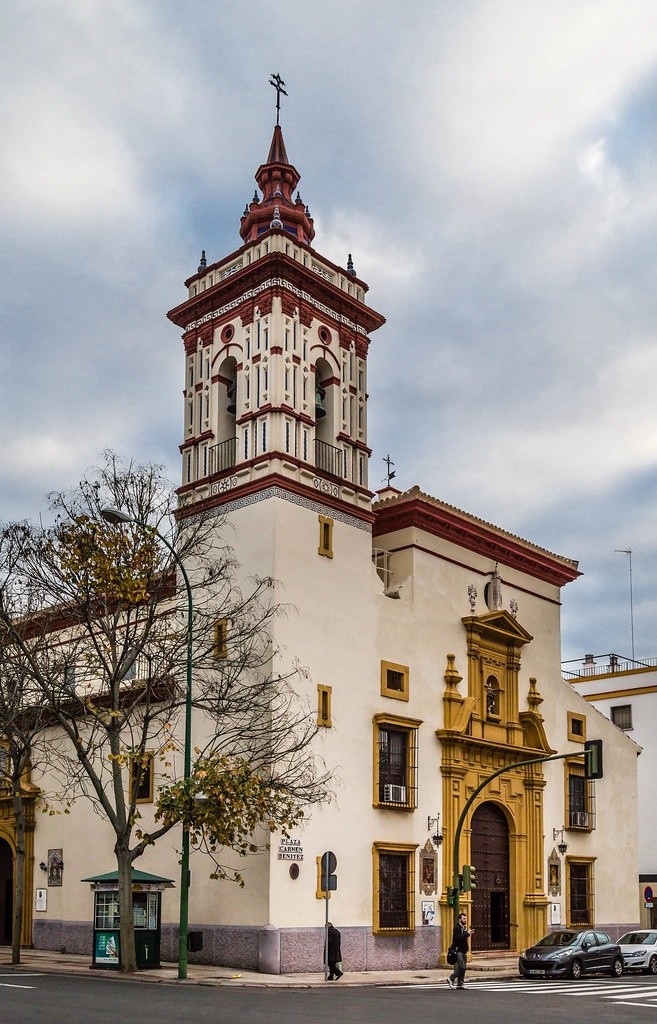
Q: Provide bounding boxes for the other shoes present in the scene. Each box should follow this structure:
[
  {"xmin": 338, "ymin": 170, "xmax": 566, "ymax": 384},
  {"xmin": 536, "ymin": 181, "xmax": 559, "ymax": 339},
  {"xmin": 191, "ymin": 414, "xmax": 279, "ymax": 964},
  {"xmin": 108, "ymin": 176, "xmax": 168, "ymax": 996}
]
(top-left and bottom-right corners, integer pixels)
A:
[
  {"xmin": 335, "ymin": 972, "xmax": 344, "ymax": 981},
  {"xmin": 327, "ymin": 975, "xmax": 334, "ymax": 980}
]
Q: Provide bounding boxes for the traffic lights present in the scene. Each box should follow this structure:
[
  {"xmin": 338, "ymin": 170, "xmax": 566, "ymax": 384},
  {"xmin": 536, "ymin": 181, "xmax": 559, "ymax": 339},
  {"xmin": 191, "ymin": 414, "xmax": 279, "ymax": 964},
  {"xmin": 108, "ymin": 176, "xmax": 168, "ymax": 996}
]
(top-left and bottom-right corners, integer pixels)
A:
[
  {"xmin": 452, "ymin": 873, "xmax": 463, "ymax": 891},
  {"xmin": 463, "ymin": 864, "xmax": 476, "ymax": 892},
  {"xmin": 448, "ymin": 887, "xmax": 459, "ymax": 908}
]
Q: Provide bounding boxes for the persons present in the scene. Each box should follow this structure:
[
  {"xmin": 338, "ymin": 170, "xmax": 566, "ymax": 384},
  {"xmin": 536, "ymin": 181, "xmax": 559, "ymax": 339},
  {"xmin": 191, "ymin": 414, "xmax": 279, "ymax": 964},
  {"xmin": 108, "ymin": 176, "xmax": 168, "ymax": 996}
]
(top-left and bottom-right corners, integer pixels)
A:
[
  {"xmin": 446, "ymin": 913, "xmax": 475, "ymax": 990},
  {"xmin": 323, "ymin": 922, "xmax": 344, "ymax": 981}
]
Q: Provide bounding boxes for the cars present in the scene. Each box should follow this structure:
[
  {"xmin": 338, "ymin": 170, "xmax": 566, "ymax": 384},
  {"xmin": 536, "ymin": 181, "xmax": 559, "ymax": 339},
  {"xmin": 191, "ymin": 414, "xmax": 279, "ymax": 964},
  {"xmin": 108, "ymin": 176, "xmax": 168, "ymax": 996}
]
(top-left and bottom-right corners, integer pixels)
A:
[
  {"xmin": 616, "ymin": 929, "xmax": 657, "ymax": 975},
  {"xmin": 519, "ymin": 928, "xmax": 625, "ymax": 979}
]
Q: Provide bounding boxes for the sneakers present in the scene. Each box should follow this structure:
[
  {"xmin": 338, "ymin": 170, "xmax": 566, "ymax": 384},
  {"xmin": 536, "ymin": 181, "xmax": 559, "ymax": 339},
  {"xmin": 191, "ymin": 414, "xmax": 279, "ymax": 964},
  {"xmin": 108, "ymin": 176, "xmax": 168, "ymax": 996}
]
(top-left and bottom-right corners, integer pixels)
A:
[
  {"xmin": 447, "ymin": 977, "xmax": 456, "ymax": 990},
  {"xmin": 457, "ymin": 985, "xmax": 468, "ymax": 989}
]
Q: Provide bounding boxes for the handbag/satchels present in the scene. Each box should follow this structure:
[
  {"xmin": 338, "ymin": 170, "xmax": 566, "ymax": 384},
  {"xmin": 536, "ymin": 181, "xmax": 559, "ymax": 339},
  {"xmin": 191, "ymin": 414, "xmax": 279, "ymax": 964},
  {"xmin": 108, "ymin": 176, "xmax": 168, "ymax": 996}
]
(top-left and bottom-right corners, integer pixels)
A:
[{"xmin": 447, "ymin": 944, "xmax": 458, "ymax": 965}]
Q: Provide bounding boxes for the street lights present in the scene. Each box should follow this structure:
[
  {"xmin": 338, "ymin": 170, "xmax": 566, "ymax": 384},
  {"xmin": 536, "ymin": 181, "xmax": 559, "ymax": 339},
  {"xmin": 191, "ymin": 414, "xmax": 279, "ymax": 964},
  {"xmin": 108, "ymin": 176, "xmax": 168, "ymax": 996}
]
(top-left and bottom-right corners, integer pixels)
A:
[{"xmin": 97, "ymin": 508, "xmax": 193, "ymax": 981}]
